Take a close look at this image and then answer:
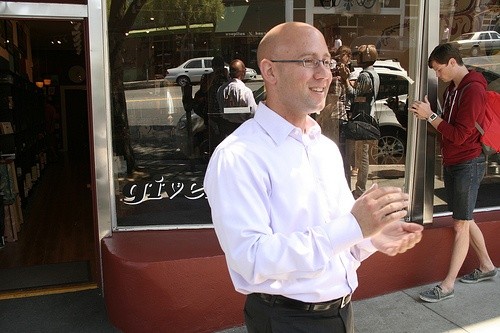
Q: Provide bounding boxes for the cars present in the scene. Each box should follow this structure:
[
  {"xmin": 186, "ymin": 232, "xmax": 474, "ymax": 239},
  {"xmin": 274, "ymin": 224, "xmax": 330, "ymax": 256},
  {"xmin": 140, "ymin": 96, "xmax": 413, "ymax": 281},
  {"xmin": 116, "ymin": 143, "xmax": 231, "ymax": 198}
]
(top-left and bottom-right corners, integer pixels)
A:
[
  {"xmin": 165, "ymin": 57, "xmax": 257, "ymax": 86},
  {"xmin": 448, "ymin": 31, "xmax": 500, "ymax": 57}
]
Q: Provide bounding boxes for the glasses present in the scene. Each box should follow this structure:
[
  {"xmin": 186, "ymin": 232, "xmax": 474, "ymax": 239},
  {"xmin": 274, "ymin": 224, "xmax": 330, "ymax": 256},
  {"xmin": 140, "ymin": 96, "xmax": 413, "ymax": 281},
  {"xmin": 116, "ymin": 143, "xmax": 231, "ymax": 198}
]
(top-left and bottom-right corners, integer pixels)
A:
[{"xmin": 271, "ymin": 56, "xmax": 336, "ymax": 70}]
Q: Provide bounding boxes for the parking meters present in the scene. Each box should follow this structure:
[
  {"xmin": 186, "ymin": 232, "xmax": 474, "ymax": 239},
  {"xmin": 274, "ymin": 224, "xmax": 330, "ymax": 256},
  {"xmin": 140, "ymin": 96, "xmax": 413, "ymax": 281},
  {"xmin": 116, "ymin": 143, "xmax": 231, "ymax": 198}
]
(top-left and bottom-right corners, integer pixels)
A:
[{"xmin": 181, "ymin": 81, "xmax": 195, "ymax": 177}]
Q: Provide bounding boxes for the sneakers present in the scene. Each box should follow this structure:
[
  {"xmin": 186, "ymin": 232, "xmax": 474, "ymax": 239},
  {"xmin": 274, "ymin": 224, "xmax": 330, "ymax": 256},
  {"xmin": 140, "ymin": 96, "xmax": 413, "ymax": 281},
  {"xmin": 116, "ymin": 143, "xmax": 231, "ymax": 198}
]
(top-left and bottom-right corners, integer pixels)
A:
[
  {"xmin": 419, "ymin": 285, "xmax": 455, "ymax": 303},
  {"xmin": 460, "ymin": 266, "xmax": 498, "ymax": 283}
]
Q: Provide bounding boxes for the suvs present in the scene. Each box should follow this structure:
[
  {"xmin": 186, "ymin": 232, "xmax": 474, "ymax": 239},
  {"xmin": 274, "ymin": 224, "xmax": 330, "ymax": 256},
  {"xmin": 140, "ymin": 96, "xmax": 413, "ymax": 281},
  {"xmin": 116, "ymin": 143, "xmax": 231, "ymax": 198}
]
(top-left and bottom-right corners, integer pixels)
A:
[{"xmin": 175, "ymin": 60, "xmax": 500, "ymax": 184}]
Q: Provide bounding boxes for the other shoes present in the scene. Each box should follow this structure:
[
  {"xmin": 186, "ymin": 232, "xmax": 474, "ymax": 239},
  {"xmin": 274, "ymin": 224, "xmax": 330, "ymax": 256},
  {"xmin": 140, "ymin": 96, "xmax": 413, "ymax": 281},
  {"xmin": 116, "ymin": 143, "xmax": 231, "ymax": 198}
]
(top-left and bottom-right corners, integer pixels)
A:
[{"xmin": 352, "ymin": 190, "xmax": 360, "ymax": 200}]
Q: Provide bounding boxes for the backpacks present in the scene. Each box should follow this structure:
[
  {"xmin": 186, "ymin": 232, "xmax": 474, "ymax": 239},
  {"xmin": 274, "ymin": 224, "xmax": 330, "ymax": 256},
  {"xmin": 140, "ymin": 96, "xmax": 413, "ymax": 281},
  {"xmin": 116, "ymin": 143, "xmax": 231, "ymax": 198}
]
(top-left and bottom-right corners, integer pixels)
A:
[{"xmin": 458, "ymin": 82, "xmax": 500, "ymax": 156}]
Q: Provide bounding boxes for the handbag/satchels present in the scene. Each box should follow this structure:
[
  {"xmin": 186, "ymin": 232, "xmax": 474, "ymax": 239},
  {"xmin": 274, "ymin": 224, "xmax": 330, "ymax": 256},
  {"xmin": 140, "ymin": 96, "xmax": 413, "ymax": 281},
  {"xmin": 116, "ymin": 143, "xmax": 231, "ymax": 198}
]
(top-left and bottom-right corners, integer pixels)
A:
[{"xmin": 345, "ymin": 112, "xmax": 381, "ymax": 140}]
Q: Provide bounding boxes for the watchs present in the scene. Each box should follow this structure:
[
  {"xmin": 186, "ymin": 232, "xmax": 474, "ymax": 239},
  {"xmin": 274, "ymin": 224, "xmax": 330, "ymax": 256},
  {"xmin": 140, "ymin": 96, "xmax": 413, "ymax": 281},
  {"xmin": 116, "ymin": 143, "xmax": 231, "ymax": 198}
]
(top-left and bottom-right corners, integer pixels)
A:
[{"xmin": 428, "ymin": 113, "xmax": 438, "ymax": 123}]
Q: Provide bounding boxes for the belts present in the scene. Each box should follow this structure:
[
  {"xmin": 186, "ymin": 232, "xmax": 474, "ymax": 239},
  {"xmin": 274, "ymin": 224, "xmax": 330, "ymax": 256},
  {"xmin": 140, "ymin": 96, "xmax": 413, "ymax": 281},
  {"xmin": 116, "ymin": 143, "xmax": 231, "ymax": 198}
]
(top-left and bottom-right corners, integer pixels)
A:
[{"xmin": 253, "ymin": 292, "xmax": 352, "ymax": 313}]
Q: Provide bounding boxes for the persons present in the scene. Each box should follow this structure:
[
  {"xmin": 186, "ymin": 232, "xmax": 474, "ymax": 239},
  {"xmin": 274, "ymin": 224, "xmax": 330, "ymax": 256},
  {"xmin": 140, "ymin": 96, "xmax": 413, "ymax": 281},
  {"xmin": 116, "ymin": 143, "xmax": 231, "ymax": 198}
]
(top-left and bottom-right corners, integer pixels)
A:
[
  {"xmin": 204, "ymin": 22, "xmax": 423, "ymax": 333},
  {"xmin": 441, "ymin": 26, "xmax": 449, "ymax": 43},
  {"xmin": 409, "ymin": 43, "xmax": 497, "ymax": 302},
  {"xmin": 315, "ymin": 45, "xmax": 408, "ymax": 199},
  {"xmin": 194, "ymin": 57, "xmax": 257, "ymax": 153}
]
